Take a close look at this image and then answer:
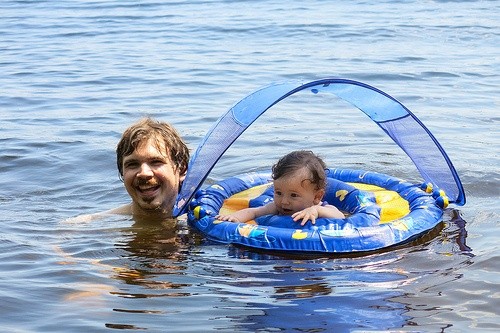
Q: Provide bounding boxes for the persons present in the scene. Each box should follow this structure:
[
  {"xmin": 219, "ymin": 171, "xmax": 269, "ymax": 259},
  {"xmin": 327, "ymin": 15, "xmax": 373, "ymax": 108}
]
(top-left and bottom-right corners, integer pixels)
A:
[
  {"xmin": 92, "ymin": 118, "xmax": 189, "ymax": 219},
  {"xmin": 214, "ymin": 150, "xmax": 344, "ymax": 225}
]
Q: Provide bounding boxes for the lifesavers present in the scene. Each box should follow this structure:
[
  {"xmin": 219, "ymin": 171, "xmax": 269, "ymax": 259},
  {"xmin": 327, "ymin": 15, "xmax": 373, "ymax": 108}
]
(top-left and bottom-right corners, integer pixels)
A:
[{"xmin": 186, "ymin": 167, "xmax": 449, "ymax": 260}]
